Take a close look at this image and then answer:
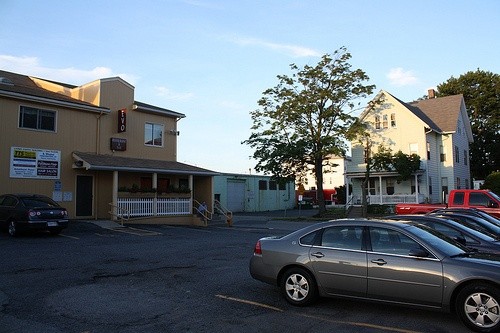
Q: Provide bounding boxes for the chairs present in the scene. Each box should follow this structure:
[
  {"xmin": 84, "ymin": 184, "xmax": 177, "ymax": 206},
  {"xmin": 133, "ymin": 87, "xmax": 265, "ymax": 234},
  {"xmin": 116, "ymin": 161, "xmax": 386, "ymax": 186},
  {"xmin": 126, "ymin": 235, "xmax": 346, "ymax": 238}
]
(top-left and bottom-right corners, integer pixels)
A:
[
  {"xmin": 326, "ymin": 232, "xmax": 343, "ymax": 241},
  {"xmin": 360, "ymin": 231, "xmax": 380, "ymax": 246}
]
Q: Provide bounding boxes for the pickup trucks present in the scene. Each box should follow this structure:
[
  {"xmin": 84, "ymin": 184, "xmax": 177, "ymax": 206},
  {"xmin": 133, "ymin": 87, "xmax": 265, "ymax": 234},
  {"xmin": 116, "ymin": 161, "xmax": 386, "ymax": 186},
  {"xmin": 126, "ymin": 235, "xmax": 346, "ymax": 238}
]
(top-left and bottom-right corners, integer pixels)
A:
[{"xmin": 393, "ymin": 189, "xmax": 500, "ymax": 219}]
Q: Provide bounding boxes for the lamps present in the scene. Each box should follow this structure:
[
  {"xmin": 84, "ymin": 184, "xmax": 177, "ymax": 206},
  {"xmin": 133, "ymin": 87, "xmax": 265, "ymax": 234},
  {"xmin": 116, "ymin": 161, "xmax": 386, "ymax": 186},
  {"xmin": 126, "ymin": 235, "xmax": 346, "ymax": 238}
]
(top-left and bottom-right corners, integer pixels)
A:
[{"xmin": 297, "ymin": 194, "xmax": 303, "ymax": 216}]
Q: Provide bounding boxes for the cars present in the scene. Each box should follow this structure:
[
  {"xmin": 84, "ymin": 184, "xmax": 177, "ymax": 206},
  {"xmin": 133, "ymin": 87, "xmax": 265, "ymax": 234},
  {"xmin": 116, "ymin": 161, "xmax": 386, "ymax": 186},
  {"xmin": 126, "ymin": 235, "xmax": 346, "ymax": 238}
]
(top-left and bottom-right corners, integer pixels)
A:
[
  {"xmin": 0, "ymin": 193, "xmax": 71, "ymax": 236},
  {"xmin": 249, "ymin": 217, "xmax": 500, "ymax": 333},
  {"xmin": 377, "ymin": 207, "xmax": 500, "ymax": 256}
]
